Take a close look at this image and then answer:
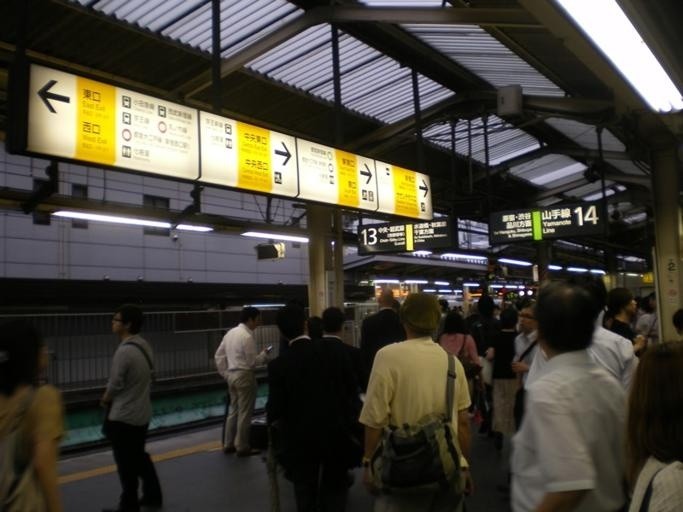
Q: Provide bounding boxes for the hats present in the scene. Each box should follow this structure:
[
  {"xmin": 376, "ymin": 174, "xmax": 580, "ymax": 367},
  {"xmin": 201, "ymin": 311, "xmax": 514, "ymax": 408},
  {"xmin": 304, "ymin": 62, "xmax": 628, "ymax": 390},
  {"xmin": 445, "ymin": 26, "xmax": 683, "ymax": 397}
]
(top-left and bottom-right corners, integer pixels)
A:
[{"xmin": 401, "ymin": 293, "xmax": 440, "ymax": 333}]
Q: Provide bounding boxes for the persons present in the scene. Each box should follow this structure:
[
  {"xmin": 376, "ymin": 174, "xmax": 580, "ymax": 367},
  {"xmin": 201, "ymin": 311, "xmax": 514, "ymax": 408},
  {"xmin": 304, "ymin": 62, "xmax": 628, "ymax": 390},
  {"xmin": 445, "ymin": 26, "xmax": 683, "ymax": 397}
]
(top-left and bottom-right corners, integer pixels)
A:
[
  {"xmin": 0, "ymin": 317, "xmax": 65, "ymax": 512},
  {"xmin": 267, "ymin": 271, "xmax": 682, "ymax": 510},
  {"xmin": 215, "ymin": 307, "xmax": 269, "ymax": 458},
  {"xmin": 100, "ymin": 305, "xmax": 163, "ymax": 512}
]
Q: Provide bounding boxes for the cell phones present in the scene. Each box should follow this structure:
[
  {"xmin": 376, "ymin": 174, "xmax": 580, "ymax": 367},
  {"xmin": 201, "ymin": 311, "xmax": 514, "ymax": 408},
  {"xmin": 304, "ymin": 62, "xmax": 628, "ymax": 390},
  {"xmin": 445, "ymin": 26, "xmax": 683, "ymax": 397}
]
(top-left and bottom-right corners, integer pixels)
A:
[{"xmin": 266, "ymin": 345, "xmax": 272, "ymax": 353}]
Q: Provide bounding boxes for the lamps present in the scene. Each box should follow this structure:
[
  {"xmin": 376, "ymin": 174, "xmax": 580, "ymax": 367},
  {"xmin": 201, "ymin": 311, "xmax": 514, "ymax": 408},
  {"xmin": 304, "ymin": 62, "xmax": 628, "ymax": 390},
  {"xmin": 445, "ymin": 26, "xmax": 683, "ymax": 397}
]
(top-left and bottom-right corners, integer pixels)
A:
[{"xmin": 553, "ymin": 0, "xmax": 683, "ymax": 122}]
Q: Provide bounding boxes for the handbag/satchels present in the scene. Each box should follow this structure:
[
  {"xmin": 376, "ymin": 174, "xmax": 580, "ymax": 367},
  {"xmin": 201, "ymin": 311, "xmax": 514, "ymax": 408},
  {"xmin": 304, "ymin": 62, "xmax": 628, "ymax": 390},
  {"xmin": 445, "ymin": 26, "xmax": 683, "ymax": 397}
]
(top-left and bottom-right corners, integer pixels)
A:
[
  {"xmin": 2, "ymin": 428, "xmax": 58, "ymax": 512},
  {"xmin": 369, "ymin": 413, "xmax": 460, "ymax": 491},
  {"xmin": 459, "ymin": 348, "xmax": 483, "ymax": 379}
]
